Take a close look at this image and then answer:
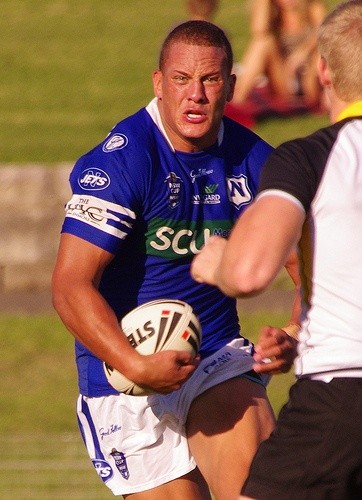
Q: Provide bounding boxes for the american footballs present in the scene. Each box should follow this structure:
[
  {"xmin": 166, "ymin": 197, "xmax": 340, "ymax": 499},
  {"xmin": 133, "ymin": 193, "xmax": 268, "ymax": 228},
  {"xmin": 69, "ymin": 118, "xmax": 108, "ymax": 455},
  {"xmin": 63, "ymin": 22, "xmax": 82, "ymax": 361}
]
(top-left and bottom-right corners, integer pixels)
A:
[{"xmin": 102, "ymin": 297, "xmax": 203, "ymax": 398}]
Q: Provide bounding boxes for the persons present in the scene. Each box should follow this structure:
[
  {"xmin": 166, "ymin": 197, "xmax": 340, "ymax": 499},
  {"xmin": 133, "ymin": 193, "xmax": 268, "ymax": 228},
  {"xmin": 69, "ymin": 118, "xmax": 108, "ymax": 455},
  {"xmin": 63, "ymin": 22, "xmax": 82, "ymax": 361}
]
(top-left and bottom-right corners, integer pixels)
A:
[
  {"xmin": 187, "ymin": 0, "xmax": 327, "ymax": 136},
  {"xmin": 51, "ymin": 21, "xmax": 307, "ymax": 500},
  {"xmin": 190, "ymin": 0, "xmax": 362, "ymax": 500}
]
